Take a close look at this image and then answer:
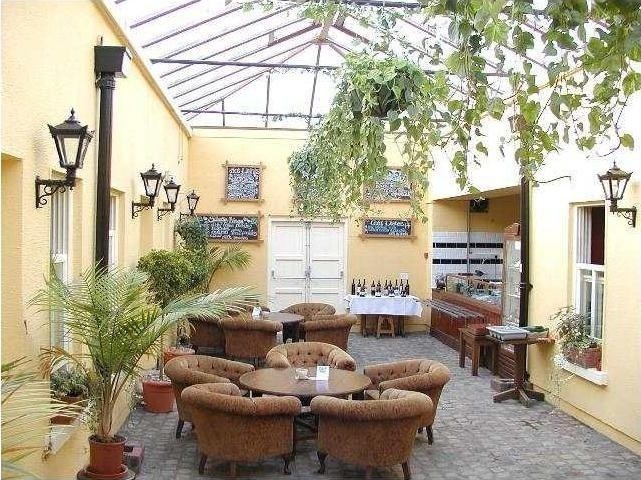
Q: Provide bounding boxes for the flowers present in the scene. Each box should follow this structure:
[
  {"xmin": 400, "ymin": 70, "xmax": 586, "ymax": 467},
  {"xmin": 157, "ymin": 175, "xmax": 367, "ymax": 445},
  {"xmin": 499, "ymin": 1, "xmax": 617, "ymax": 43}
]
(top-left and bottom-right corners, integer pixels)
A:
[{"xmin": 544, "ymin": 302, "xmax": 601, "ymax": 419}]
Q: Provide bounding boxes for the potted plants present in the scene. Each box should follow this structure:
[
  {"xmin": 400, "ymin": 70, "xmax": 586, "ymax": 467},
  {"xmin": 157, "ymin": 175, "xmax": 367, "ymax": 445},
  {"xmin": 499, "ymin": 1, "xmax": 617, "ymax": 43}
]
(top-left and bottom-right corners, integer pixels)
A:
[
  {"xmin": 49, "ymin": 361, "xmax": 87, "ymax": 425},
  {"xmin": 133, "ymin": 247, "xmax": 214, "ymax": 413},
  {"xmin": 26, "ymin": 252, "xmax": 261, "ymax": 480},
  {"xmin": 158, "ymin": 243, "xmax": 253, "ymax": 366},
  {"xmin": 330, "ymin": 37, "xmax": 450, "ymax": 241},
  {"xmin": 172, "ymin": 213, "xmax": 209, "ymax": 255},
  {"xmin": 286, "ymin": 139, "xmax": 343, "ymax": 233}
]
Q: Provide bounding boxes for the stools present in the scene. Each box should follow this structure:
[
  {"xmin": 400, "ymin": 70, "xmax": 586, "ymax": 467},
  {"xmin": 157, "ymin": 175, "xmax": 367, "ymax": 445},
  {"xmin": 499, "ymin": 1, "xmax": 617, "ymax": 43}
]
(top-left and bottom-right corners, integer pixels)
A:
[{"xmin": 375, "ymin": 313, "xmax": 397, "ymax": 340}]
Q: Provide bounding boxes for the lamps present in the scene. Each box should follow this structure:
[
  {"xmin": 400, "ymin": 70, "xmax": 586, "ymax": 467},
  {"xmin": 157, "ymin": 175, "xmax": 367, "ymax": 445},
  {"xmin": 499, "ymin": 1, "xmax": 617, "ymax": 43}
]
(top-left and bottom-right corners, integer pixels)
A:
[
  {"xmin": 179, "ymin": 188, "xmax": 200, "ymax": 221},
  {"xmin": 131, "ymin": 161, "xmax": 165, "ymax": 218},
  {"xmin": 32, "ymin": 104, "xmax": 97, "ymax": 209},
  {"xmin": 156, "ymin": 176, "xmax": 181, "ymax": 220},
  {"xmin": 595, "ymin": 158, "xmax": 637, "ymax": 229}
]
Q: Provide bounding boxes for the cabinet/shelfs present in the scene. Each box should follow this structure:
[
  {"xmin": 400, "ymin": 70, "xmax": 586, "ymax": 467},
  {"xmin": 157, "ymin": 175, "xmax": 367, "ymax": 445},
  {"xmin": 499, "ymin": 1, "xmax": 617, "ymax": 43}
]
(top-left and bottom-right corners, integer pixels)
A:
[{"xmin": 498, "ymin": 222, "xmax": 522, "ymax": 381}]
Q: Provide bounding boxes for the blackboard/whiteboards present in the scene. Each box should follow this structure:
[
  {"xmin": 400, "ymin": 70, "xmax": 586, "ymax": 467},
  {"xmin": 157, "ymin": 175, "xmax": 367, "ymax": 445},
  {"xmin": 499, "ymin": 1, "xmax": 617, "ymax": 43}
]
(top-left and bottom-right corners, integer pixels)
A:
[
  {"xmin": 181, "ymin": 213, "xmax": 260, "ymax": 244},
  {"xmin": 362, "ymin": 217, "xmax": 412, "ymax": 238},
  {"xmin": 224, "ymin": 165, "xmax": 262, "ymax": 203},
  {"xmin": 363, "ymin": 165, "xmax": 413, "ymax": 203}
]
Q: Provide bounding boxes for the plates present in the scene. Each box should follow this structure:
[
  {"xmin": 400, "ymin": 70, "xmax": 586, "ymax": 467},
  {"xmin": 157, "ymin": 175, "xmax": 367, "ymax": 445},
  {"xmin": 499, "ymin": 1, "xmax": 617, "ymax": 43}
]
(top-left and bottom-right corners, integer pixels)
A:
[{"xmin": 295, "ymin": 376, "xmax": 309, "ymax": 380}]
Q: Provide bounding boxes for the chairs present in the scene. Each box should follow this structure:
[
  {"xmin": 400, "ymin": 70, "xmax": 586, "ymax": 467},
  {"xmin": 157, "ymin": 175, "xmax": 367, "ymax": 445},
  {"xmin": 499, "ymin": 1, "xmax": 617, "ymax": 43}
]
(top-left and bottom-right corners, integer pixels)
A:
[
  {"xmin": 309, "ymin": 388, "xmax": 433, "ymax": 478},
  {"xmin": 279, "ymin": 302, "xmax": 336, "ymax": 344},
  {"xmin": 166, "ymin": 354, "xmax": 255, "ymax": 440},
  {"xmin": 217, "ymin": 301, "xmax": 256, "ymax": 313},
  {"xmin": 180, "ymin": 383, "xmax": 302, "ymax": 477},
  {"xmin": 303, "ymin": 312, "xmax": 358, "ymax": 351},
  {"xmin": 265, "ymin": 341, "xmax": 357, "ymax": 371},
  {"xmin": 217, "ymin": 316, "xmax": 283, "ymax": 361},
  {"xmin": 176, "ymin": 307, "xmax": 242, "ymax": 354},
  {"xmin": 364, "ymin": 358, "xmax": 451, "ymax": 445}
]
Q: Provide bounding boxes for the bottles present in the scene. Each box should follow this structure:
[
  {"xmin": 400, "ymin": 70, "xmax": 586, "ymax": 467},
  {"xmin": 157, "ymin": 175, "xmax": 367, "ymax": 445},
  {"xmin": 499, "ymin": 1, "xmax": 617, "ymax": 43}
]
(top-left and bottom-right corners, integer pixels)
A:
[
  {"xmin": 351, "ymin": 278, "xmax": 411, "ymax": 298},
  {"xmin": 256, "ymin": 303, "xmax": 262, "ymax": 310}
]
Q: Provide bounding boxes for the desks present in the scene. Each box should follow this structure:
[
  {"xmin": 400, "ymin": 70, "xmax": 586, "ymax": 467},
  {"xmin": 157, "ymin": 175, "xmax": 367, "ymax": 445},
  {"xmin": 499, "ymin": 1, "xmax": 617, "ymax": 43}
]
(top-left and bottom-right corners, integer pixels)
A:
[
  {"xmin": 239, "ymin": 366, "xmax": 372, "ymax": 452},
  {"xmin": 344, "ymin": 294, "xmax": 422, "ymax": 338},
  {"xmin": 457, "ymin": 328, "xmax": 498, "ymax": 376},
  {"xmin": 238, "ymin": 311, "xmax": 304, "ymax": 341},
  {"xmin": 484, "ymin": 333, "xmax": 556, "ymax": 409}
]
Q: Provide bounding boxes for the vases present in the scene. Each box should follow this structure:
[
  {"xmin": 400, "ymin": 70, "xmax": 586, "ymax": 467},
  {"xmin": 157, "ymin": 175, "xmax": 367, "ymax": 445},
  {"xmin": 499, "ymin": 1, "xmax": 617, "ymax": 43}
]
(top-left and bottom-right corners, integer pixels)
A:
[{"xmin": 562, "ymin": 339, "xmax": 601, "ymax": 371}]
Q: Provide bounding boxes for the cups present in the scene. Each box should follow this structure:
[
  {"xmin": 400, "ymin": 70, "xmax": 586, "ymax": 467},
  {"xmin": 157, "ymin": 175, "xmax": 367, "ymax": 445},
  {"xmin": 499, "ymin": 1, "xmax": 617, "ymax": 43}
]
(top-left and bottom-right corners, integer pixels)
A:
[
  {"xmin": 261, "ymin": 311, "xmax": 269, "ymax": 317},
  {"xmin": 296, "ymin": 367, "xmax": 309, "ymax": 379}
]
